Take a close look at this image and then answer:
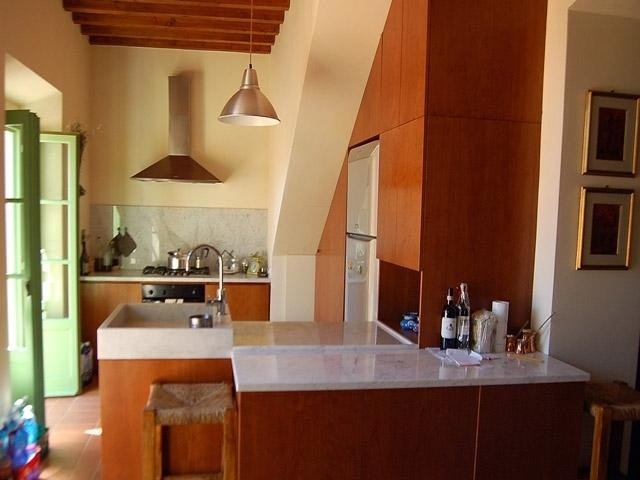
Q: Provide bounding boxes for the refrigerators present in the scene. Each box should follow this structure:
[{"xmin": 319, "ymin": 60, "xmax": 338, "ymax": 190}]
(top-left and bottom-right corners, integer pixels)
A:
[
  {"xmin": 342, "ymin": 138, "xmax": 378, "ymax": 319},
  {"xmin": 340, "ymin": 321, "xmax": 379, "ymax": 379}
]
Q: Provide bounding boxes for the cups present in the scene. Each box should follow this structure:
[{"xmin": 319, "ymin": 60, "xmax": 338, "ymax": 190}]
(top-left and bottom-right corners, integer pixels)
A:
[
  {"xmin": 507, "ymin": 328, "xmax": 537, "ymax": 354},
  {"xmin": 217, "ymin": 255, "xmax": 264, "ymax": 274}
]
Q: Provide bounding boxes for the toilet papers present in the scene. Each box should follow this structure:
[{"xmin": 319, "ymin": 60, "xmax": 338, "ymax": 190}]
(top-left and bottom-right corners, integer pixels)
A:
[{"xmin": 491, "ymin": 301, "xmax": 510, "ymax": 354}]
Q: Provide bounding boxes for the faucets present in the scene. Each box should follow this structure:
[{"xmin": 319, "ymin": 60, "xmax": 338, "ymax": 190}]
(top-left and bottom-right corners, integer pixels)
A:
[{"xmin": 185, "ymin": 244, "xmax": 228, "ymax": 316}]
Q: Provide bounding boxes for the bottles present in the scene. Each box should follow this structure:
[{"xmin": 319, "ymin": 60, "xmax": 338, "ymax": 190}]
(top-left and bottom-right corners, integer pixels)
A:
[
  {"xmin": 80, "ymin": 234, "xmax": 112, "ymax": 277},
  {"xmin": 399, "ymin": 312, "xmax": 419, "ymax": 334},
  {"xmin": 1, "ymin": 393, "xmax": 38, "ymax": 479},
  {"xmin": 189, "ymin": 313, "xmax": 214, "ymax": 328},
  {"xmin": 440, "ymin": 288, "xmax": 456, "ymax": 351},
  {"xmin": 456, "ymin": 284, "xmax": 471, "ymax": 351}
]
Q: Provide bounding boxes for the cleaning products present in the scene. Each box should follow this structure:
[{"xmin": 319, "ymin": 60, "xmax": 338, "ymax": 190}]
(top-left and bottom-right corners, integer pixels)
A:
[{"xmin": 1, "ymin": 394, "xmax": 50, "ymax": 478}]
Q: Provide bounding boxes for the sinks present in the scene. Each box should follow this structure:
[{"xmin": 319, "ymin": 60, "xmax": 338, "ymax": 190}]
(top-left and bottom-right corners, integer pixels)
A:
[{"xmin": 94, "ymin": 302, "xmax": 235, "ymax": 360}]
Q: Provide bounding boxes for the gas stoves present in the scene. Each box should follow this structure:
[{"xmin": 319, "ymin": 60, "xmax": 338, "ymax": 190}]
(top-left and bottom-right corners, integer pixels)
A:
[{"xmin": 141, "ymin": 265, "xmax": 210, "ymax": 298}]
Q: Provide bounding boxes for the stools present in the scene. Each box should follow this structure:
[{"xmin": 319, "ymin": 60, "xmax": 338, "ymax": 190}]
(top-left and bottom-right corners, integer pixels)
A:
[
  {"xmin": 583, "ymin": 380, "xmax": 640, "ymax": 480},
  {"xmin": 142, "ymin": 381, "xmax": 237, "ymax": 480}
]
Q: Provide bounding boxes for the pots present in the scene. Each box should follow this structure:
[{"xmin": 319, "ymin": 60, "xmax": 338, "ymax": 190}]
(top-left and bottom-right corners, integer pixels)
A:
[{"xmin": 164, "ymin": 247, "xmax": 192, "ymax": 270}]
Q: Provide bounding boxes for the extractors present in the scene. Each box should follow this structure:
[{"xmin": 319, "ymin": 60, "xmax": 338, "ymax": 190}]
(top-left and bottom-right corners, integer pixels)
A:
[{"xmin": 130, "ymin": 75, "xmax": 221, "ymax": 183}]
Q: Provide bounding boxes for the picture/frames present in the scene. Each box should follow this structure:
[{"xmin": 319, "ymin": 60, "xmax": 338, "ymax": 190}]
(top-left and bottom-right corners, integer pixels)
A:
[
  {"xmin": 576, "ymin": 186, "xmax": 635, "ymax": 271},
  {"xmin": 581, "ymin": 89, "xmax": 640, "ymax": 178}
]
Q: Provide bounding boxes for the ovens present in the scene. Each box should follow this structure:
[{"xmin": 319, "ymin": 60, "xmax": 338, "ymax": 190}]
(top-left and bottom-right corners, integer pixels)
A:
[{"xmin": 142, "ymin": 298, "xmax": 203, "ymax": 303}]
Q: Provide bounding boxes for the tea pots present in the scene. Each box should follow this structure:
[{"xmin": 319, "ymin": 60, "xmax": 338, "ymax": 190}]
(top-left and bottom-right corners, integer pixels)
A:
[{"xmin": 191, "ymin": 247, "xmax": 209, "ymax": 268}]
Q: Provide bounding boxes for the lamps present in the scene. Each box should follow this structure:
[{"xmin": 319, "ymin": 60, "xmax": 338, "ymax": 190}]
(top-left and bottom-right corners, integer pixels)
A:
[{"xmin": 217, "ymin": 0, "xmax": 281, "ymax": 128}]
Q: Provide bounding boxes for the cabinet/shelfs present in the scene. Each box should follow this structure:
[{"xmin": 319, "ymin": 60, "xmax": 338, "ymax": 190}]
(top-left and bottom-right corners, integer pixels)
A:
[
  {"xmin": 376, "ymin": 115, "xmax": 541, "ymax": 272},
  {"xmin": 378, "ymin": 0, "xmax": 548, "ymax": 134},
  {"xmin": 78, "ymin": 281, "xmax": 141, "ymax": 369},
  {"xmin": 204, "ymin": 283, "xmax": 270, "ymax": 321}
]
